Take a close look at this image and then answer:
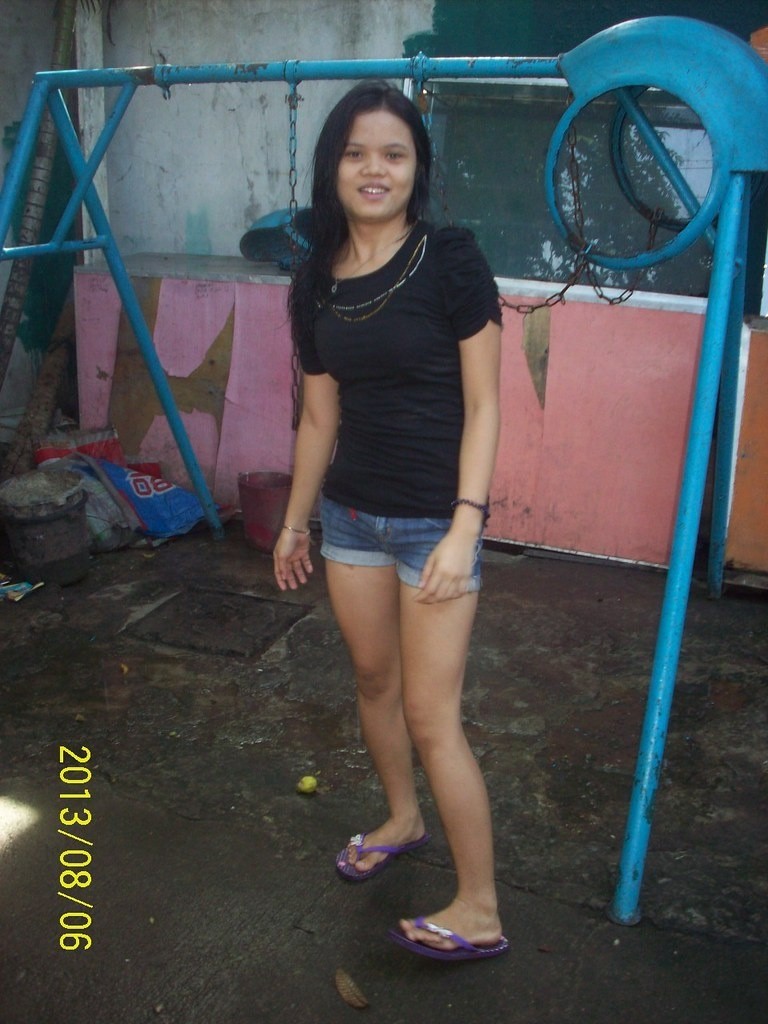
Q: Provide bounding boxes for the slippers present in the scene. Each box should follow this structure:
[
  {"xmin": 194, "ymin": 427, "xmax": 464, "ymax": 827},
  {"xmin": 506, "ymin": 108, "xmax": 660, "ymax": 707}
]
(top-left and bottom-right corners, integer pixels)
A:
[
  {"xmin": 387, "ymin": 915, "xmax": 510, "ymax": 960},
  {"xmin": 336, "ymin": 832, "xmax": 429, "ymax": 880}
]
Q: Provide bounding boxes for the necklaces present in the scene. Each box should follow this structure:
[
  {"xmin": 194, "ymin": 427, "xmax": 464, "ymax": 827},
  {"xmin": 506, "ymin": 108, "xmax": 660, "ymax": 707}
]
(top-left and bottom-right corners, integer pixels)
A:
[{"xmin": 323, "ymin": 221, "xmax": 427, "ymax": 322}]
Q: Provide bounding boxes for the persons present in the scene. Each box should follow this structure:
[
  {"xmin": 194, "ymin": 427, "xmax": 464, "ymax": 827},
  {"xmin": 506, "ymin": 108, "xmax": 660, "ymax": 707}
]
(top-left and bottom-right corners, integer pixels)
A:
[{"xmin": 273, "ymin": 82, "xmax": 511, "ymax": 961}]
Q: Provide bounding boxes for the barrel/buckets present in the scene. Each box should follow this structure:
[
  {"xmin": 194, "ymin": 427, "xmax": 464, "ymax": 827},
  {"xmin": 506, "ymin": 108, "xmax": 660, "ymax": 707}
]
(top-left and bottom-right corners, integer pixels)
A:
[
  {"xmin": 0, "ymin": 488, "xmax": 92, "ymax": 585},
  {"xmin": 238, "ymin": 471, "xmax": 293, "ymax": 553}
]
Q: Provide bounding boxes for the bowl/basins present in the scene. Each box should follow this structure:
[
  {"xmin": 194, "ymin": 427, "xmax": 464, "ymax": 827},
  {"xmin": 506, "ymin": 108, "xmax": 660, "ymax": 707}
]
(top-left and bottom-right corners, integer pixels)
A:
[{"xmin": 0, "ymin": 468, "xmax": 87, "ymax": 514}]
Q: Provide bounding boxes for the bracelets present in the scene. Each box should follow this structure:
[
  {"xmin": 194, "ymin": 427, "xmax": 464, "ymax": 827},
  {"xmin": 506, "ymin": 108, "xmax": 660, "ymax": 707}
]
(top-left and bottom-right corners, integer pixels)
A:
[
  {"xmin": 451, "ymin": 499, "xmax": 490, "ymax": 528},
  {"xmin": 283, "ymin": 525, "xmax": 310, "ymax": 535}
]
